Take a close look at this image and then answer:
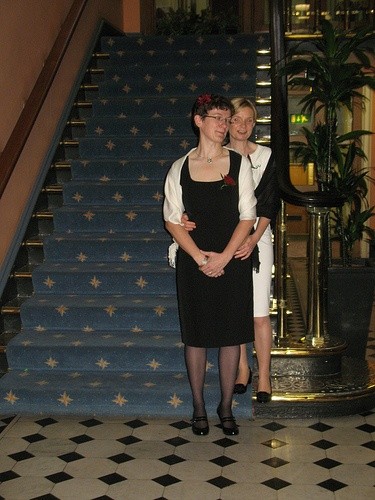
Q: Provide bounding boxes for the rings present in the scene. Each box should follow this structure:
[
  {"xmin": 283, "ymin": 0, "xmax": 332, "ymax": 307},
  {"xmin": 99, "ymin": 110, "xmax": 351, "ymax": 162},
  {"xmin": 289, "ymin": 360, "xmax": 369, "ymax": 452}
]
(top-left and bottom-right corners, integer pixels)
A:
[{"xmin": 210, "ymin": 272, "xmax": 212, "ymax": 274}]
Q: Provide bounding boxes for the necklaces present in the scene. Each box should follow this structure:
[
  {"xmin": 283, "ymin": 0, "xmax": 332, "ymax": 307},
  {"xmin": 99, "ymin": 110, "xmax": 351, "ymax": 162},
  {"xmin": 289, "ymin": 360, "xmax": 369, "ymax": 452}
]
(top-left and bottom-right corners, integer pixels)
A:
[{"xmin": 195, "ymin": 150, "xmax": 223, "ymax": 163}]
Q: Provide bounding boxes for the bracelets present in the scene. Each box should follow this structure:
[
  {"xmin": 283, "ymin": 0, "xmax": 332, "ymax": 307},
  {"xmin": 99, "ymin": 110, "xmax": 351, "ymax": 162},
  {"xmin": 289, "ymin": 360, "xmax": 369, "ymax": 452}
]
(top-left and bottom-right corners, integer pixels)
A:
[{"xmin": 202, "ymin": 256, "xmax": 208, "ymax": 264}]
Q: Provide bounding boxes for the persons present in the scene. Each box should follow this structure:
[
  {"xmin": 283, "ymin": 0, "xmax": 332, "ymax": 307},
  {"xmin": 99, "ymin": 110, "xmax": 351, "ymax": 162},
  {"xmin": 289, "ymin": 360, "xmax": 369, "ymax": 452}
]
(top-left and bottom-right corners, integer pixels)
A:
[
  {"xmin": 179, "ymin": 97, "xmax": 280, "ymax": 404},
  {"xmin": 162, "ymin": 93, "xmax": 259, "ymax": 436}
]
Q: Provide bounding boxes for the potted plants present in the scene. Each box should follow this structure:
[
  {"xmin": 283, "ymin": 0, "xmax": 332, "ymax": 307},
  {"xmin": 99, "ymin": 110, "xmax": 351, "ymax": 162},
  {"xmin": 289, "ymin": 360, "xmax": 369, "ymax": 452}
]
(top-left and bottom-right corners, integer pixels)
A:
[{"xmin": 269, "ymin": 14, "xmax": 375, "ymax": 357}]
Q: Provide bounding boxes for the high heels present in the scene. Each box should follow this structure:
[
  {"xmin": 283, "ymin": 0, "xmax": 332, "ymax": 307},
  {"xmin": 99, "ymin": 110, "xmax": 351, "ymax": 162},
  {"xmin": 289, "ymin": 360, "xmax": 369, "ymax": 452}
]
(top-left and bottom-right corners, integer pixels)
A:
[
  {"xmin": 256, "ymin": 378, "xmax": 272, "ymax": 402},
  {"xmin": 234, "ymin": 367, "xmax": 252, "ymax": 395},
  {"xmin": 192, "ymin": 413, "xmax": 209, "ymax": 435},
  {"xmin": 218, "ymin": 405, "xmax": 240, "ymax": 435}
]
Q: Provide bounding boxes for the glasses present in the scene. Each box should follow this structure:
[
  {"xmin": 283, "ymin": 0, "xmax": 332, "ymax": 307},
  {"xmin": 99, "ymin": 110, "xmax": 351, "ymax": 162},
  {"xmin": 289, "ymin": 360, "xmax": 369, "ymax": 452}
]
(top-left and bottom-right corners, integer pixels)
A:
[
  {"xmin": 206, "ymin": 115, "xmax": 234, "ymax": 124},
  {"xmin": 233, "ymin": 117, "xmax": 255, "ymax": 124}
]
